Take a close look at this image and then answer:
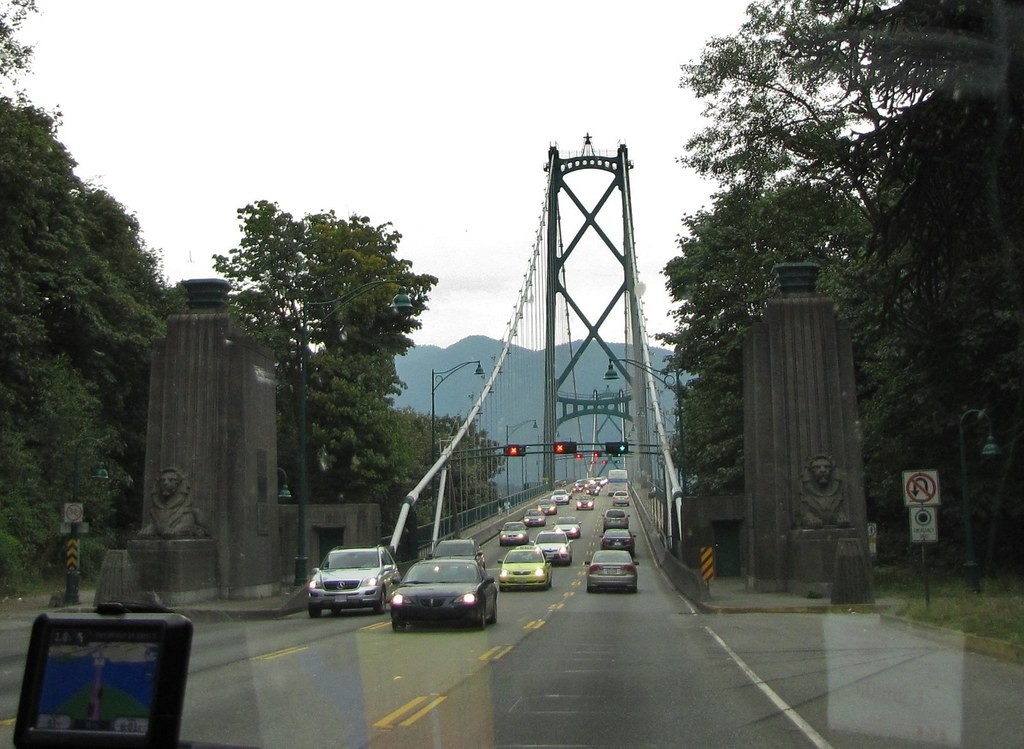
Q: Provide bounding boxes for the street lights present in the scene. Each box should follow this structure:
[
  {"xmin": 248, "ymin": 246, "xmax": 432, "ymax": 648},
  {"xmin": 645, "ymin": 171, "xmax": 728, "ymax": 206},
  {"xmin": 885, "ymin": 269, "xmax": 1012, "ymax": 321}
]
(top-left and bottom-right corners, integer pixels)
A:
[
  {"xmin": 602, "ymin": 358, "xmax": 687, "ymax": 493},
  {"xmin": 431, "ymin": 361, "xmax": 486, "ymax": 522},
  {"xmin": 293, "ymin": 279, "xmax": 413, "ymax": 586},
  {"xmin": 506, "ymin": 419, "xmax": 538, "ymax": 496},
  {"xmin": 960, "ymin": 409, "xmax": 1003, "ymax": 591},
  {"xmin": 65, "ymin": 437, "xmax": 108, "ymax": 606}
]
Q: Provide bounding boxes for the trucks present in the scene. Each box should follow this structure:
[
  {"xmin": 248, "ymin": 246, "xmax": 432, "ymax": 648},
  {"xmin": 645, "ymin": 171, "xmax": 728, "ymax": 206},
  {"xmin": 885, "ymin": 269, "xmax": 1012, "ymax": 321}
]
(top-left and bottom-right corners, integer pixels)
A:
[{"xmin": 607, "ymin": 468, "xmax": 628, "ymax": 496}]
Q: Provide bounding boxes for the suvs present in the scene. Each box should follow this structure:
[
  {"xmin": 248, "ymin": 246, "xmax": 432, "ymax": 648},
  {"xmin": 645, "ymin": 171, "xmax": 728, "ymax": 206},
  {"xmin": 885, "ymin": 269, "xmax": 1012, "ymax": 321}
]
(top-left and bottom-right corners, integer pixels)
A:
[{"xmin": 307, "ymin": 544, "xmax": 402, "ymax": 618}]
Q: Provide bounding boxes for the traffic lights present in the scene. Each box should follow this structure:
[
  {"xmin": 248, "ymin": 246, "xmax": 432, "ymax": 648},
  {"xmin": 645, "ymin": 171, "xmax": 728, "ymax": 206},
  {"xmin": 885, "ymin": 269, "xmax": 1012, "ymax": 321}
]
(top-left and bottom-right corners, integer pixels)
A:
[
  {"xmin": 606, "ymin": 441, "xmax": 628, "ymax": 454},
  {"xmin": 613, "ymin": 454, "xmax": 621, "ymax": 457},
  {"xmin": 575, "ymin": 454, "xmax": 582, "ymax": 458},
  {"xmin": 594, "ymin": 451, "xmax": 601, "ymax": 457},
  {"xmin": 553, "ymin": 442, "xmax": 578, "ymax": 454},
  {"xmin": 508, "ymin": 445, "xmax": 519, "ymax": 456}
]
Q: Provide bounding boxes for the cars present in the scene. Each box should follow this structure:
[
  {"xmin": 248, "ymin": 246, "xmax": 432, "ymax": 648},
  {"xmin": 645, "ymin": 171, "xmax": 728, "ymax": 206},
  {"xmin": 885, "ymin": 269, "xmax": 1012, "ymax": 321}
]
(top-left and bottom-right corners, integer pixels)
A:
[
  {"xmin": 390, "ymin": 560, "xmax": 499, "ymax": 630},
  {"xmin": 601, "ymin": 508, "xmax": 630, "ymax": 533},
  {"xmin": 599, "ymin": 529, "xmax": 636, "ymax": 556},
  {"xmin": 498, "ymin": 548, "xmax": 552, "ymax": 591},
  {"xmin": 536, "ymin": 499, "xmax": 557, "ymax": 515},
  {"xmin": 612, "ymin": 491, "xmax": 630, "ymax": 506},
  {"xmin": 523, "ymin": 509, "xmax": 546, "ymax": 526},
  {"xmin": 531, "ymin": 529, "xmax": 573, "ymax": 565},
  {"xmin": 551, "ymin": 516, "xmax": 582, "ymax": 540},
  {"xmin": 585, "ymin": 550, "xmax": 639, "ymax": 592},
  {"xmin": 499, "ymin": 521, "xmax": 529, "ymax": 546},
  {"xmin": 428, "ymin": 539, "xmax": 486, "ymax": 569},
  {"xmin": 576, "ymin": 495, "xmax": 595, "ymax": 510},
  {"xmin": 550, "ymin": 476, "xmax": 608, "ymax": 505}
]
